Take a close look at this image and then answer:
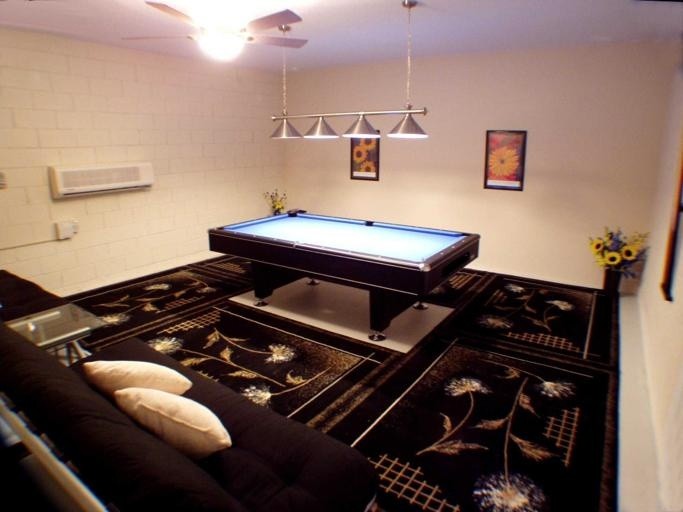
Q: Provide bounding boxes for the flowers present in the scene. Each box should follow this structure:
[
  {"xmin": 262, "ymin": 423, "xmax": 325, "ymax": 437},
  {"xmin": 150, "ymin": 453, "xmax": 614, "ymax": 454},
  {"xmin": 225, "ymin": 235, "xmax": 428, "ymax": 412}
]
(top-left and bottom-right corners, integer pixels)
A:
[
  {"xmin": 262, "ymin": 189, "xmax": 288, "ymax": 215},
  {"xmin": 588, "ymin": 225, "xmax": 651, "ymax": 278}
]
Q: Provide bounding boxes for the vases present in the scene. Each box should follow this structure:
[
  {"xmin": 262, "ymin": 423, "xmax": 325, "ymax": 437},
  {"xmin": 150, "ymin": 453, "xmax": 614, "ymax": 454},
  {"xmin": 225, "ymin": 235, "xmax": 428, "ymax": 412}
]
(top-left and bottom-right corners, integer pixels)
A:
[{"xmin": 601, "ymin": 268, "xmax": 621, "ymax": 297}]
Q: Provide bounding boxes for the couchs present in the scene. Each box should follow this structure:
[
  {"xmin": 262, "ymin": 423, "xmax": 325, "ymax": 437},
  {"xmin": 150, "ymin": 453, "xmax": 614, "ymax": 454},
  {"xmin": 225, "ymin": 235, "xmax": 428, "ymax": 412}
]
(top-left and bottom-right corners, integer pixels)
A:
[
  {"xmin": 1, "ymin": 323, "xmax": 379, "ymax": 512},
  {"xmin": 0, "ymin": 269, "xmax": 68, "ymax": 324}
]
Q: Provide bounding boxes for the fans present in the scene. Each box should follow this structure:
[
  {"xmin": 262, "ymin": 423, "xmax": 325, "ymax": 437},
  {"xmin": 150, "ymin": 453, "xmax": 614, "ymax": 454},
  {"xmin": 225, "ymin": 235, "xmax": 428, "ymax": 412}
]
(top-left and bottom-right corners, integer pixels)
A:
[{"xmin": 119, "ymin": 0, "xmax": 309, "ymax": 48}]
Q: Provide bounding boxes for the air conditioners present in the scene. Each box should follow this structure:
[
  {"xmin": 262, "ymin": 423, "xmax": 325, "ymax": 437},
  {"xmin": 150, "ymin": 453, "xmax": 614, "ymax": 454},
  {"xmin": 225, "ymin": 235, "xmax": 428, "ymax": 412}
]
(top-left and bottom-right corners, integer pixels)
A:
[{"xmin": 47, "ymin": 161, "xmax": 155, "ymax": 201}]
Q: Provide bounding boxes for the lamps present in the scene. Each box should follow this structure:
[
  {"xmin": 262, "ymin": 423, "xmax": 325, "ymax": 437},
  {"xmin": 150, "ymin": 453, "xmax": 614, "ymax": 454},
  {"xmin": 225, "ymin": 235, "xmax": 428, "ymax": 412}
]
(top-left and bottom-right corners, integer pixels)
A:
[{"xmin": 269, "ymin": 0, "xmax": 428, "ymax": 140}]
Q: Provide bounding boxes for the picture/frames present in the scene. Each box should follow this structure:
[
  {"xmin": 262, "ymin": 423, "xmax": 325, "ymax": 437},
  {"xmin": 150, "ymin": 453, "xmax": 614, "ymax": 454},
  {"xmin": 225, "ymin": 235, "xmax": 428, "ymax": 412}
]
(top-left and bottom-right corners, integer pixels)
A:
[
  {"xmin": 483, "ymin": 129, "xmax": 528, "ymax": 191},
  {"xmin": 350, "ymin": 130, "xmax": 380, "ymax": 182}
]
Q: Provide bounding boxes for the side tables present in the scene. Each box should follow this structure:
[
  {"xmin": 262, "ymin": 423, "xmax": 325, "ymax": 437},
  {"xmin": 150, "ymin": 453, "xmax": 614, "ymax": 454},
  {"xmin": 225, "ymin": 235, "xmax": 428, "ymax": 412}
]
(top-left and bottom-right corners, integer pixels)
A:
[{"xmin": 5, "ymin": 303, "xmax": 107, "ymax": 366}]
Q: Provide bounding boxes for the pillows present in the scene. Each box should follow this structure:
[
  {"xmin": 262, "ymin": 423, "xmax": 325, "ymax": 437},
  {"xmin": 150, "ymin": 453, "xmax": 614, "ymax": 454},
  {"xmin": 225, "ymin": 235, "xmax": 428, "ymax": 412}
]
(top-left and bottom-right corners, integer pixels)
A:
[
  {"xmin": 81, "ymin": 361, "xmax": 194, "ymax": 401},
  {"xmin": 113, "ymin": 387, "xmax": 233, "ymax": 460}
]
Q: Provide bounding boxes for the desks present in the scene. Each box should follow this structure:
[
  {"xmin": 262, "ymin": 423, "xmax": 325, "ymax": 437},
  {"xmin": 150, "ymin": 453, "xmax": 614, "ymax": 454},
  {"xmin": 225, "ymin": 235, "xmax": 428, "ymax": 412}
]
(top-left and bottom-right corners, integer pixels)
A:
[{"xmin": 208, "ymin": 209, "xmax": 480, "ymax": 341}]
